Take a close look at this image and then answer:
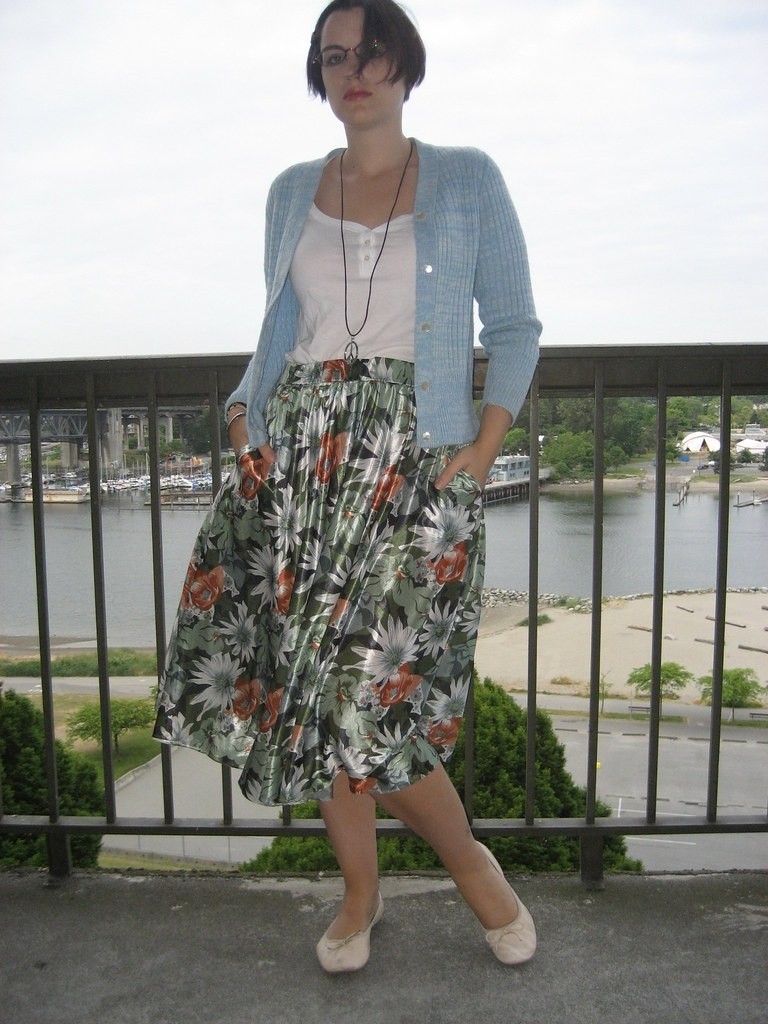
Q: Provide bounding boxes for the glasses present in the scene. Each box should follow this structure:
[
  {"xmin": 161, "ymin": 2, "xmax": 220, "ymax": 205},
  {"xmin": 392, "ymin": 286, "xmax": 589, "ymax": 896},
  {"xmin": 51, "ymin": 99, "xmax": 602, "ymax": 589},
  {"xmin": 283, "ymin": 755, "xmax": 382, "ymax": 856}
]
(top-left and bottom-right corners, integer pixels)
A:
[{"xmin": 313, "ymin": 39, "xmax": 389, "ymax": 67}]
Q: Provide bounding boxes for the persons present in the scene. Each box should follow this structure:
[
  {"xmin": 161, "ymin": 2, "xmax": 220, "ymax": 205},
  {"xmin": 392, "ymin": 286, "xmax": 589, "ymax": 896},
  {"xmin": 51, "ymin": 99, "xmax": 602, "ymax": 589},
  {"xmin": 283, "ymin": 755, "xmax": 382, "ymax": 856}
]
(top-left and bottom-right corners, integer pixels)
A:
[{"xmin": 152, "ymin": 0, "xmax": 542, "ymax": 971}]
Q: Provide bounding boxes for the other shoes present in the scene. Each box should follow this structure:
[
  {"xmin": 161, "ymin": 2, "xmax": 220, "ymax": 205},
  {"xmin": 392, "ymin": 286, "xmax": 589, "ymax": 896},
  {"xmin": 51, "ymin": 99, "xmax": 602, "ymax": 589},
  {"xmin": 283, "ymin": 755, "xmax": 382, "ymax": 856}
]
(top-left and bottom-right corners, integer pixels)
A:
[
  {"xmin": 315, "ymin": 890, "xmax": 385, "ymax": 973},
  {"xmin": 474, "ymin": 841, "xmax": 538, "ymax": 964}
]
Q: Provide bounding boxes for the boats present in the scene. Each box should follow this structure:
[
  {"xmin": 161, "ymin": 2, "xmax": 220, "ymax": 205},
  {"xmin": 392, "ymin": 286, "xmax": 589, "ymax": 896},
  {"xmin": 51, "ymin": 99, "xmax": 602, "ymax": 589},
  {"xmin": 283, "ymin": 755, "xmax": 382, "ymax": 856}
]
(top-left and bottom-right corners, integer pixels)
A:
[{"xmin": 0, "ymin": 453, "xmax": 232, "ymax": 498}]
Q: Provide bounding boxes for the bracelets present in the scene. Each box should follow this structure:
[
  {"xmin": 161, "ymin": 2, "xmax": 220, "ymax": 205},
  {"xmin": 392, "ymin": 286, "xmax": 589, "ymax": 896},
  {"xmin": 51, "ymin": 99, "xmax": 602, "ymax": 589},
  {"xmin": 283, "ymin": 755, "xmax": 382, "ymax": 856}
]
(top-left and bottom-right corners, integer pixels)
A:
[{"xmin": 225, "ymin": 410, "xmax": 246, "ymax": 435}]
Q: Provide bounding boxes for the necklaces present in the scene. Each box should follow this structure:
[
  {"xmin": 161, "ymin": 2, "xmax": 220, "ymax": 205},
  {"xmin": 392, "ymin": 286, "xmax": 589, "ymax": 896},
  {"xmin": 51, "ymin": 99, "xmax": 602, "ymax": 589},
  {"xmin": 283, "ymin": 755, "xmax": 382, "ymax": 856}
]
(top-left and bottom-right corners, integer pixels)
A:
[{"xmin": 340, "ymin": 139, "xmax": 413, "ymax": 366}]
